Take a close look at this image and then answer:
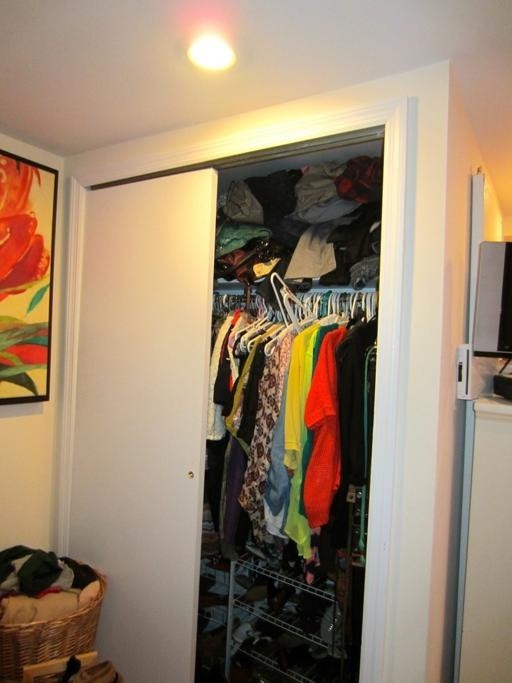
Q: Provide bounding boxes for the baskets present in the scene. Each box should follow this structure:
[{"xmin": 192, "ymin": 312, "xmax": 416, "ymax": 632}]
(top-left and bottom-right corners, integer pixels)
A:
[{"xmin": 0, "ymin": 559, "xmax": 107, "ymax": 683}]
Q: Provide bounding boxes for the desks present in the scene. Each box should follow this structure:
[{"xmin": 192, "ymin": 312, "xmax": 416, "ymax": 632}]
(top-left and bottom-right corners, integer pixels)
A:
[{"xmin": 458, "ymin": 397, "xmax": 512, "ymax": 682}]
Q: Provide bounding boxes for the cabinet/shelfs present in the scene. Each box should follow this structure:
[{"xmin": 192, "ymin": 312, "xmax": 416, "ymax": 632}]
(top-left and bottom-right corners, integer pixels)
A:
[{"xmin": 77, "ymin": 142, "xmax": 381, "ymax": 682}]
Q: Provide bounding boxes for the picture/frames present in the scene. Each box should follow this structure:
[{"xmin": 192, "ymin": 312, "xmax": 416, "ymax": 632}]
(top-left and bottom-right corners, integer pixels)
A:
[{"xmin": 0, "ymin": 147, "xmax": 59, "ymax": 405}]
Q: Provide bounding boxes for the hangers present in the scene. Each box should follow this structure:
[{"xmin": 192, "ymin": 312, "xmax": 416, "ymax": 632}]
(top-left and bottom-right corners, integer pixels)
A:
[{"xmin": 215, "ymin": 277, "xmax": 376, "ymax": 357}]
